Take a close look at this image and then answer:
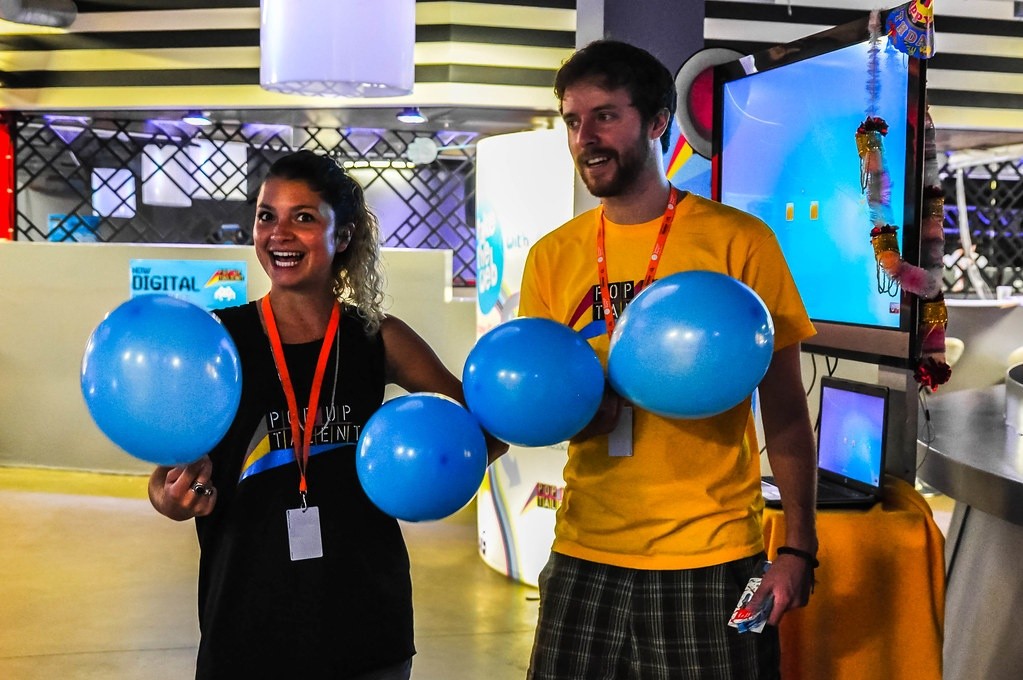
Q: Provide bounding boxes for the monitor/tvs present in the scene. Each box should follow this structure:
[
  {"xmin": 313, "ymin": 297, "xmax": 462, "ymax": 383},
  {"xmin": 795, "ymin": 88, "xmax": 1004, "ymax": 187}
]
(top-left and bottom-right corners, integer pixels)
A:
[{"xmin": 710, "ymin": 0, "xmax": 928, "ymax": 369}]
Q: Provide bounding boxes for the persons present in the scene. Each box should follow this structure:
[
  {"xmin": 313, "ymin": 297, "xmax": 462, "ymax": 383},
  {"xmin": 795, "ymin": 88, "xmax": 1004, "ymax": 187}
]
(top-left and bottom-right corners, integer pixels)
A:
[
  {"xmin": 146, "ymin": 151, "xmax": 508, "ymax": 680},
  {"xmin": 517, "ymin": 40, "xmax": 819, "ymax": 680}
]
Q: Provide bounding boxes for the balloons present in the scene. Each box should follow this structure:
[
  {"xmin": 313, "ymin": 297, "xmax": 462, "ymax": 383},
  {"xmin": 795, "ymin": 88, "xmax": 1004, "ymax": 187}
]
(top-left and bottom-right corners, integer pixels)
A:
[
  {"xmin": 462, "ymin": 318, "xmax": 605, "ymax": 447},
  {"xmin": 80, "ymin": 293, "xmax": 242, "ymax": 466},
  {"xmin": 607, "ymin": 270, "xmax": 776, "ymax": 420},
  {"xmin": 355, "ymin": 391, "xmax": 489, "ymax": 523}
]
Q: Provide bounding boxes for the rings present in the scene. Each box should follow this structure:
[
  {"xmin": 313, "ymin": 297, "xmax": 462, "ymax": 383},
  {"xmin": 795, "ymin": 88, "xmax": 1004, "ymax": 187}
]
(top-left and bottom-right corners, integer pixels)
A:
[
  {"xmin": 190, "ymin": 481, "xmax": 206, "ymax": 495},
  {"xmin": 203, "ymin": 486, "xmax": 213, "ymax": 496}
]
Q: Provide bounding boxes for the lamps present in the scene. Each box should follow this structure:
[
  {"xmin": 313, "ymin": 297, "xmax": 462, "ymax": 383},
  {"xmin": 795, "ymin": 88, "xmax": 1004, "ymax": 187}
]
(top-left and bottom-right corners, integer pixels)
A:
[
  {"xmin": 260, "ymin": 0, "xmax": 416, "ymax": 99},
  {"xmin": 396, "ymin": 107, "xmax": 428, "ymax": 125},
  {"xmin": 182, "ymin": 111, "xmax": 213, "ymax": 127}
]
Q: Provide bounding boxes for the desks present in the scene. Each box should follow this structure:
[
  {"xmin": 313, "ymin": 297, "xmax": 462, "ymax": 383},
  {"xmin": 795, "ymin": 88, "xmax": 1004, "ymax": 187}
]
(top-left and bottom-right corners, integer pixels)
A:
[
  {"xmin": 761, "ymin": 477, "xmax": 945, "ymax": 680},
  {"xmin": 909, "ymin": 385, "xmax": 1023, "ymax": 680}
]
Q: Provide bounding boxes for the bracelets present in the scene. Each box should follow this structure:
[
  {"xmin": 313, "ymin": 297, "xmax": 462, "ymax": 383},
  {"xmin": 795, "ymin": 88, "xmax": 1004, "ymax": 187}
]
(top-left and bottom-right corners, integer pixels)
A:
[{"xmin": 777, "ymin": 546, "xmax": 819, "ymax": 569}]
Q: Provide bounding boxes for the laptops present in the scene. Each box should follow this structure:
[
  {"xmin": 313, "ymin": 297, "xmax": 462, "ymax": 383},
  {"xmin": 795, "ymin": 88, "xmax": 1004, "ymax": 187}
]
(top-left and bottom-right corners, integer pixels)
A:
[{"xmin": 761, "ymin": 376, "xmax": 890, "ymax": 510}]
